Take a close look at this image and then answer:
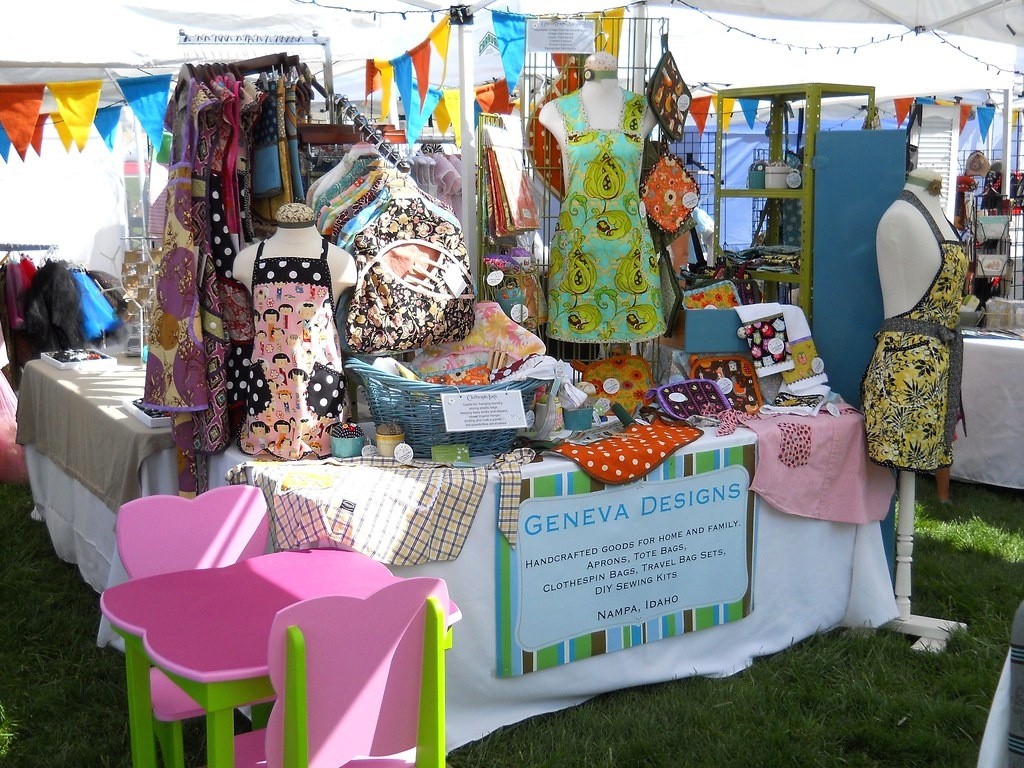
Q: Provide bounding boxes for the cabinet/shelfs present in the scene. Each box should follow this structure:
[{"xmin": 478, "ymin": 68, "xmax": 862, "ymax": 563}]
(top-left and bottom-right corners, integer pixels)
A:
[{"xmin": 712, "ymin": 83, "xmax": 877, "ymax": 324}]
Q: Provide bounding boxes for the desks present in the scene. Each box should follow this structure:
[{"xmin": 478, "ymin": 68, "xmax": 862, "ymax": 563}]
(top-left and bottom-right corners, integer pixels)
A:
[
  {"xmin": 209, "ymin": 406, "xmax": 895, "ymax": 754},
  {"xmin": 16, "ymin": 346, "xmax": 181, "ymax": 593},
  {"xmin": 926, "ymin": 329, "xmax": 1024, "ymax": 493},
  {"xmin": 100, "ymin": 549, "xmax": 461, "ymax": 767}
]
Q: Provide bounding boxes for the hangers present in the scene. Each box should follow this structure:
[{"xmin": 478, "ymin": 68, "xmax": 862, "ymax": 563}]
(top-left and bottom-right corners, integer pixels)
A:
[
  {"xmin": 176, "ymin": 27, "xmax": 457, "ymax": 205},
  {"xmin": 0, "ymin": 243, "xmax": 91, "ymax": 273}
]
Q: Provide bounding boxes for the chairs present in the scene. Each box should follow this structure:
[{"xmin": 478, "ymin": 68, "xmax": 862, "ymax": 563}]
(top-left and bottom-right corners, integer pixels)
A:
[
  {"xmin": 231, "ymin": 576, "xmax": 450, "ymax": 767},
  {"xmin": 114, "ymin": 485, "xmax": 277, "ymax": 768}
]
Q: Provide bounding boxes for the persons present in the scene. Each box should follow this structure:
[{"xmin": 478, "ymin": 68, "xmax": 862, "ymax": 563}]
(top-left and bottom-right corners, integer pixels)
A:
[
  {"xmin": 539, "ymin": 52, "xmax": 668, "ymax": 343},
  {"xmin": 863, "ymin": 168, "xmax": 970, "ymax": 473},
  {"xmin": 230, "ymin": 203, "xmax": 357, "ymax": 461}
]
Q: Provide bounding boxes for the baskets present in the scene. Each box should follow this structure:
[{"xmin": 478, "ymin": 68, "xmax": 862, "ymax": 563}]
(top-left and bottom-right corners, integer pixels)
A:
[{"xmin": 343, "ymin": 356, "xmax": 558, "ymax": 459}]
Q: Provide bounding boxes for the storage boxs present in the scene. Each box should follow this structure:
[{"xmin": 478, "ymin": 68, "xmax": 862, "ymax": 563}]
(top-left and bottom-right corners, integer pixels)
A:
[
  {"xmin": 977, "ymin": 254, "xmax": 1010, "ymax": 276},
  {"xmin": 976, "ymin": 214, "xmax": 1012, "ymax": 239},
  {"xmin": 685, "ymin": 309, "xmax": 753, "ymax": 351},
  {"xmin": 42, "ymin": 349, "xmax": 117, "ymax": 370},
  {"xmin": 985, "ymin": 297, "xmax": 1024, "ymax": 327}
]
